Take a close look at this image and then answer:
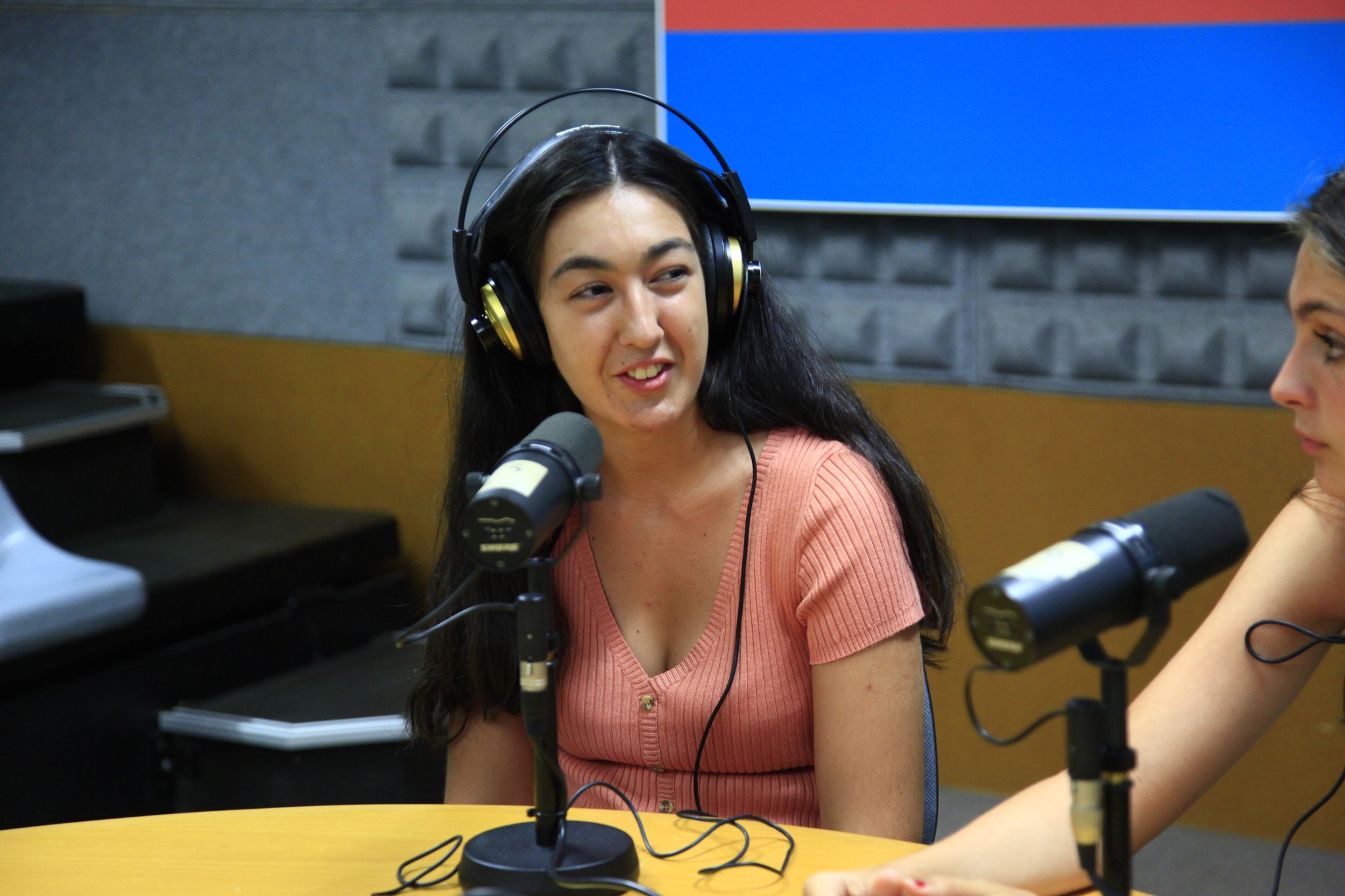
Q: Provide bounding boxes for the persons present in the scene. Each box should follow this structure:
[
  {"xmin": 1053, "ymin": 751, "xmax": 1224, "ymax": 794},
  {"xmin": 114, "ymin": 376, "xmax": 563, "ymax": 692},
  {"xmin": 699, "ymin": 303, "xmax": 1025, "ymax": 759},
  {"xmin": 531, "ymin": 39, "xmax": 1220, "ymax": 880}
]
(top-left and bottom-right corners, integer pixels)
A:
[
  {"xmin": 407, "ymin": 121, "xmax": 961, "ymax": 848},
  {"xmin": 801, "ymin": 162, "xmax": 1345, "ymax": 896}
]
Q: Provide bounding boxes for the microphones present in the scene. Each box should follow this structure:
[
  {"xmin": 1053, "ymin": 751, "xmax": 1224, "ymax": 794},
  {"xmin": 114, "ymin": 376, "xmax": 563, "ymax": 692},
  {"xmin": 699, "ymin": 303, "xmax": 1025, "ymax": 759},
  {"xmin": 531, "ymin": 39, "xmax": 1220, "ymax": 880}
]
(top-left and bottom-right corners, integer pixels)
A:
[
  {"xmin": 461, "ymin": 410, "xmax": 602, "ymax": 571},
  {"xmin": 966, "ymin": 487, "xmax": 1249, "ymax": 667}
]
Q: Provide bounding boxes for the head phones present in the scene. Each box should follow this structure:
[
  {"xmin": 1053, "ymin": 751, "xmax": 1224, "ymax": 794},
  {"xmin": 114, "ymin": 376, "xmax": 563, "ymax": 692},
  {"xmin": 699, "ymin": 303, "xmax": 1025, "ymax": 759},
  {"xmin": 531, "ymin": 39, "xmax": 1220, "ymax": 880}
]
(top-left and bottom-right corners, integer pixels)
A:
[{"xmin": 452, "ymin": 87, "xmax": 764, "ymax": 366}]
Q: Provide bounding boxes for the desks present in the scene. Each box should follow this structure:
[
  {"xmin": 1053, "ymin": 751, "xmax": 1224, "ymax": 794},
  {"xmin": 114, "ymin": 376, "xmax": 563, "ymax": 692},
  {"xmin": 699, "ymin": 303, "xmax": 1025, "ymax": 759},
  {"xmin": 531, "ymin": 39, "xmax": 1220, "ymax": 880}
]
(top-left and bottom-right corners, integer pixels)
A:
[{"xmin": 0, "ymin": 805, "xmax": 935, "ymax": 896}]
